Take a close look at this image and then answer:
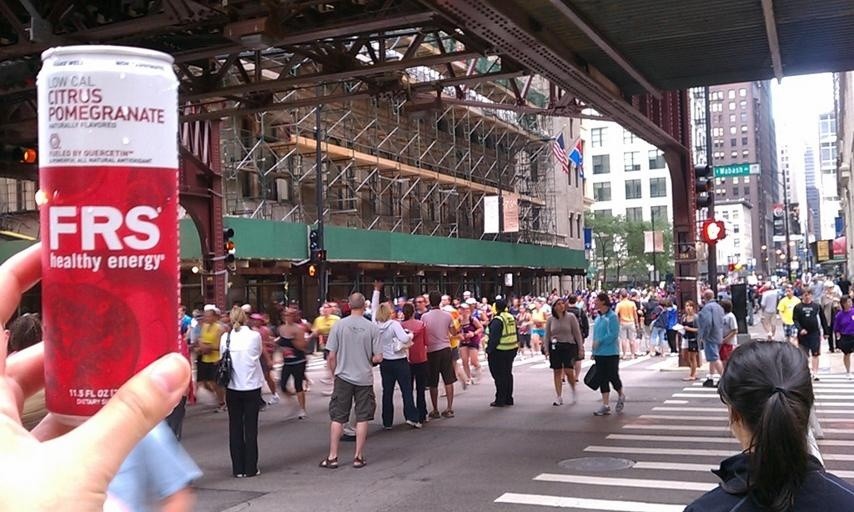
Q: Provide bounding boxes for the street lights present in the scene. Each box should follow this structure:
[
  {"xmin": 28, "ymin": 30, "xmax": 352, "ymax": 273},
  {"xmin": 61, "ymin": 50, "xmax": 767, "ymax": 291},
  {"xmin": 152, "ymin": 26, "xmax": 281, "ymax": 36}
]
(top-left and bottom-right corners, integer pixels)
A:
[{"xmin": 760, "ymin": 245, "xmax": 800, "ymax": 277}]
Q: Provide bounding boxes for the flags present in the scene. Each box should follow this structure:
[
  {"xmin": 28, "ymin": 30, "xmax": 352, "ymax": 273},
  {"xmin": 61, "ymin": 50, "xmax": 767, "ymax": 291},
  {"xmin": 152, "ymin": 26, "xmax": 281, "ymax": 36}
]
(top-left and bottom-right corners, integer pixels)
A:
[
  {"xmin": 569, "ymin": 138, "xmax": 584, "ymax": 177},
  {"xmin": 552, "ymin": 132, "xmax": 570, "ymax": 174}
]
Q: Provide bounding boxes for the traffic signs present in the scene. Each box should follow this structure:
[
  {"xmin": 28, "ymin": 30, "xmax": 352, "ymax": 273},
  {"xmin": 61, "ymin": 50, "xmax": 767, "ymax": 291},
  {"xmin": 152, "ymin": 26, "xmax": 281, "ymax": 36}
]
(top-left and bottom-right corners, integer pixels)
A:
[{"xmin": 712, "ymin": 161, "xmax": 761, "ymax": 178}]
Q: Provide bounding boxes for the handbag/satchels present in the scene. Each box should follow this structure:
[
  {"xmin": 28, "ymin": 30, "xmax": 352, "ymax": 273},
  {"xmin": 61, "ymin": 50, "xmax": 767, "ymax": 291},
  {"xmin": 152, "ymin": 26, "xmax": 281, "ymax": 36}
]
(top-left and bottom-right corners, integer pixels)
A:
[
  {"xmin": 214, "ymin": 349, "xmax": 231, "ymax": 388},
  {"xmin": 584, "ymin": 365, "xmax": 607, "ymax": 390}
]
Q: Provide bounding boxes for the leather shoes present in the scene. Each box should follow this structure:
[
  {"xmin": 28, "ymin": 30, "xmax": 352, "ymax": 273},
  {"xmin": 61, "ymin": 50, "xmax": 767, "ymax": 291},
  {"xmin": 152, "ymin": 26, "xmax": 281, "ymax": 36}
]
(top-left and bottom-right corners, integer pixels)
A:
[{"xmin": 490, "ymin": 402, "xmax": 504, "ymax": 407}]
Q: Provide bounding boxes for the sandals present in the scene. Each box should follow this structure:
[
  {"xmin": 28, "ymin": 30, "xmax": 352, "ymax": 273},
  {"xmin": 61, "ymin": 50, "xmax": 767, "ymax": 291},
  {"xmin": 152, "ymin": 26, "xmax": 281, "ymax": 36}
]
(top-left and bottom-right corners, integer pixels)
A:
[
  {"xmin": 353, "ymin": 456, "xmax": 367, "ymax": 468},
  {"xmin": 318, "ymin": 457, "xmax": 338, "ymax": 468}
]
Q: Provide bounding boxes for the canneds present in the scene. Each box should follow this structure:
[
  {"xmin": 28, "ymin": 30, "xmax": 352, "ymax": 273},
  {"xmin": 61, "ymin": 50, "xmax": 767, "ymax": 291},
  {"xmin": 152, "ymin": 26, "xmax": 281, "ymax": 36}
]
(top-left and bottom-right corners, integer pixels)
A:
[{"xmin": 38, "ymin": 44, "xmax": 183, "ymax": 427}]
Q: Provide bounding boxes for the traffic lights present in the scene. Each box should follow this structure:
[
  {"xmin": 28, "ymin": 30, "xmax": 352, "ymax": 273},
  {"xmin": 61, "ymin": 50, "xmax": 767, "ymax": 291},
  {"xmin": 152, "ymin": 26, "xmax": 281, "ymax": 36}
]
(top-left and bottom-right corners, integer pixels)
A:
[
  {"xmin": 307, "ymin": 263, "xmax": 318, "ymax": 279},
  {"xmin": 703, "ymin": 221, "xmax": 726, "ymax": 243},
  {"xmin": 313, "ymin": 249, "xmax": 327, "ymax": 260},
  {"xmin": 223, "ymin": 226, "xmax": 234, "ymax": 265},
  {"xmin": 693, "ymin": 163, "xmax": 711, "ymax": 210},
  {"xmin": 309, "ymin": 229, "xmax": 318, "ymax": 252},
  {"xmin": 3, "ymin": 146, "xmax": 38, "ymax": 166}
]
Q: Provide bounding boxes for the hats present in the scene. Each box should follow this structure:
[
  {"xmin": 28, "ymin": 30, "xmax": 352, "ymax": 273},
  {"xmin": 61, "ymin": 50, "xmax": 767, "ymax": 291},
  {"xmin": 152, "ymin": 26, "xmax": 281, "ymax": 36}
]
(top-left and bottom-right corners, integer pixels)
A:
[{"xmin": 457, "ymin": 297, "xmax": 477, "ymax": 308}]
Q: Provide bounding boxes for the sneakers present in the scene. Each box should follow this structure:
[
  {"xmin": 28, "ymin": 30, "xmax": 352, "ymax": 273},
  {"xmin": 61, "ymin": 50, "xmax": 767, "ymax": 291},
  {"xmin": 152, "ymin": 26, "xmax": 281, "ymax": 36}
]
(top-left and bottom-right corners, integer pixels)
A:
[
  {"xmin": 343, "ymin": 427, "xmax": 356, "ymax": 436},
  {"xmin": 553, "ymin": 397, "xmax": 563, "ymax": 406},
  {"xmin": 614, "ymin": 394, "xmax": 627, "ymax": 414},
  {"xmin": 593, "ymin": 405, "xmax": 611, "ymax": 415},
  {"xmin": 268, "ymin": 396, "xmax": 280, "ymax": 405},
  {"xmin": 406, "ymin": 409, "xmax": 455, "ymax": 428},
  {"xmin": 703, "ymin": 377, "xmax": 713, "ymax": 387}
]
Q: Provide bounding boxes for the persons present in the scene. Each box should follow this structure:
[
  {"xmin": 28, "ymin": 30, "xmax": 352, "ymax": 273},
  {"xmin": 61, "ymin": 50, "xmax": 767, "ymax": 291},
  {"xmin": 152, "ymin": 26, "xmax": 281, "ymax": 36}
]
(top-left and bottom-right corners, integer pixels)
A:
[
  {"xmin": 680, "ymin": 338, "xmax": 853, "ymax": 512},
  {"xmin": 0, "ymin": 240, "xmax": 190, "ymax": 512},
  {"xmin": 166, "ymin": 280, "xmax": 627, "ymax": 479},
  {"xmin": 620, "ymin": 267, "xmax": 853, "ymax": 472}
]
